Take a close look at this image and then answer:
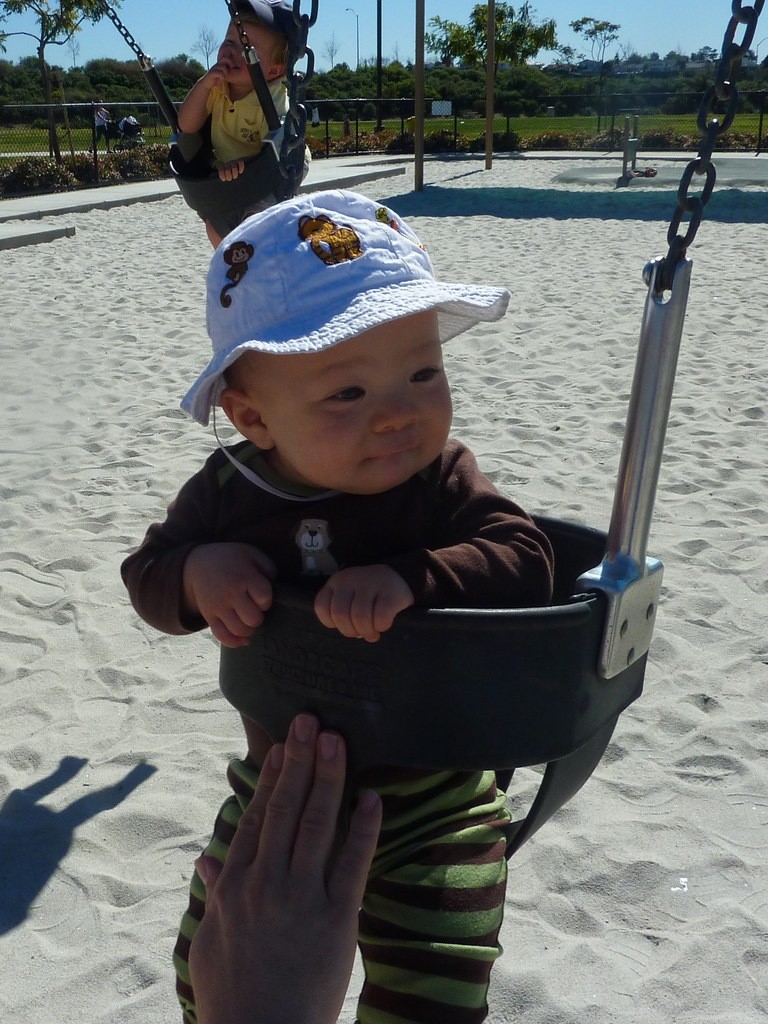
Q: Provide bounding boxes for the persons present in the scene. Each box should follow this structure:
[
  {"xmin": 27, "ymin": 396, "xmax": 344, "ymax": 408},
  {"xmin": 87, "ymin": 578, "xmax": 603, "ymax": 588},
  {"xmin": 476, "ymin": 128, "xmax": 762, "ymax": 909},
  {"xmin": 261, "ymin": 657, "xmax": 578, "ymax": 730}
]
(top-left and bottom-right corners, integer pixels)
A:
[
  {"xmin": 88, "ymin": 100, "xmax": 113, "ymax": 154},
  {"xmin": 343, "ymin": 115, "xmax": 350, "ymax": 136},
  {"xmin": 178, "ymin": 0, "xmax": 312, "ymax": 250},
  {"xmin": 375, "ymin": 120, "xmax": 386, "ymax": 132},
  {"xmin": 119, "ymin": 112, "xmax": 144, "ymax": 136},
  {"xmin": 120, "ymin": 189, "xmax": 555, "ymax": 1024},
  {"xmin": 189, "ymin": 711, "xmax": 382, "ymax": 1024}
]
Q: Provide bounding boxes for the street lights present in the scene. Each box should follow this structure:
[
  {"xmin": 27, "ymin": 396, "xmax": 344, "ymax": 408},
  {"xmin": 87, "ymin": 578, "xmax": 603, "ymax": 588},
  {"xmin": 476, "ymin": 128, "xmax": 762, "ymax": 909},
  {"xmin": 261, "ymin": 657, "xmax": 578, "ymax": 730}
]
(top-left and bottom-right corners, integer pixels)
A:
[{"xmin": 346, "ymin": 7, "xmax": 359, "ymax": 73}]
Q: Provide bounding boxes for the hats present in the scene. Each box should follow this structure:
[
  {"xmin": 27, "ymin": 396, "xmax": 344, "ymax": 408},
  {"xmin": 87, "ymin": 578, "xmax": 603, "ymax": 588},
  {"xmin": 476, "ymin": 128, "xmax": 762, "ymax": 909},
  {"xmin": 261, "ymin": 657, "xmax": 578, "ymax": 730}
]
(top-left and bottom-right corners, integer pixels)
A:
[
  {"xmin": 229, "ymin": 0, "xmax": 301, "ymax": 35},
  {"xmin": 181, "ymin": 188, "xmax": 511, "ymax": 427}
]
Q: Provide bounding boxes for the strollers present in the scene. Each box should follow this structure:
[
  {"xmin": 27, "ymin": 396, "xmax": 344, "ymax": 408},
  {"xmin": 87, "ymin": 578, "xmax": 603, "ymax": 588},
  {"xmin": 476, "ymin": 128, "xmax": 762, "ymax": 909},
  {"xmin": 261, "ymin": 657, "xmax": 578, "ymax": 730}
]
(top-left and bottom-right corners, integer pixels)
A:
[{"xmin": 106, "ymin": 117, "xmax": 147, "ymax": 153}]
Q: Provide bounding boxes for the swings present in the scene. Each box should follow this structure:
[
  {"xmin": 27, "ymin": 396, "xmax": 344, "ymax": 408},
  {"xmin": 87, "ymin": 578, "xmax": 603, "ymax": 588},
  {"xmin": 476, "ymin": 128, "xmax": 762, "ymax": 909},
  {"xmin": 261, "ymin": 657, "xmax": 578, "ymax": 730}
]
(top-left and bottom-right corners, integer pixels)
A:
[{"xmin": 88, "ymin": 0, "xmax": 766, "ymax": 864}]
